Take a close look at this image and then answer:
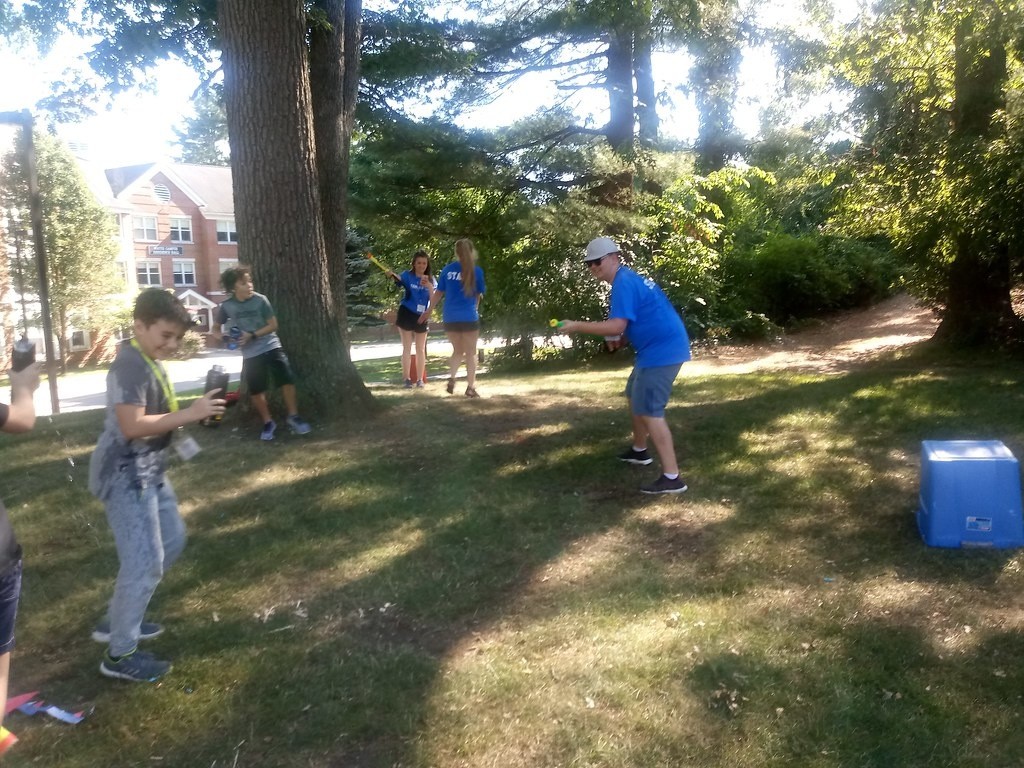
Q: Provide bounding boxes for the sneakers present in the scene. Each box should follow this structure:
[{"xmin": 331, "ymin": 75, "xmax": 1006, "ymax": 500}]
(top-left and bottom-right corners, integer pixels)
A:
[
  {"xmin": 100, "ymin": 648, "xmax": 173, "ymax": 681},
  {"xmin": 640, "ymin": 474, "xmax": 688, "ymax": 494},
  {"xmin": 91, "ymin": 622, "xmax": 162, "ymax": 643},
  {"xmin": 617, "ymin": 447, "xmax": 653, "ymax": 464},
  {"xmin": 260, "ymin": 420, "xmax": 276, "ymax": 441},
  {"xmin": 286, "ymin": 415, "xmax": 311, "ymax": 434}
]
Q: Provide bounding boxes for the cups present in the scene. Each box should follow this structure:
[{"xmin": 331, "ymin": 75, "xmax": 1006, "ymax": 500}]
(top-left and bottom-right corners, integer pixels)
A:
[{"xmin": 228, "ymin": 326, "xmax": 240, "ymax": 349}]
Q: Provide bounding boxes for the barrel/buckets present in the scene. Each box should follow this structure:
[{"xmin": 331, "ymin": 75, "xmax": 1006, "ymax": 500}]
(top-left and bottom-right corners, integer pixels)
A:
[{"xmin": 410, "ymin": 354, "xmax": 428, "ymax": 386}]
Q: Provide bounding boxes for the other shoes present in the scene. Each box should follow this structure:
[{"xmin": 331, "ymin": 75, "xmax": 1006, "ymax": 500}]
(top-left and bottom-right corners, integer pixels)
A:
[
  {"xmin": 446, "ymin": 383, "xmax": 453, "ymax": 393},
  {"xmin": 402, "ymin": 378, "xmax": 412, "ymax": 388},
  {"xmin": 415, "ymin": 380, "xmax": 423, "ymax": 387},
  {"xmin": 465, "ymin": 387, "xmax": 479, "ymax": 397}
]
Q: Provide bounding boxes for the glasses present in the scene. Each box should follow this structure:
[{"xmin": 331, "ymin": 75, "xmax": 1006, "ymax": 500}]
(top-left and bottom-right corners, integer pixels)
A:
[{"xmin": 586, "ymin": 254, "xmax": 608, "ymax": 267}]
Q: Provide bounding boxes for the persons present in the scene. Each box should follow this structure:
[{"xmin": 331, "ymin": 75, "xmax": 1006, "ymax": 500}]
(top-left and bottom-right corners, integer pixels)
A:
[
  {"xmin": 84, "ymin": 286, "xmax": 231, "ymax": 684},
  {"xmin": 385, "ymin": 238, "xmax": 486, "ymax": 397},
  {"xmin": 557, "ymin": 237, "xmax": 692, "ymax": 495},
  {"xmin": 201, "ymin": 265, "xmax": 313, "ymax": 442},
  {"xmin": 0, "ymin": 362, "xmax": 47, "ymax": 761}
]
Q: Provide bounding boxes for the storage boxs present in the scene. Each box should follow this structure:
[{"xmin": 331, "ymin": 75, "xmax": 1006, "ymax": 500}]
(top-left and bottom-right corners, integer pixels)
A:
[{"xmin": 915, "ymin": 438, "xmax": 1023, "ymax": 549}]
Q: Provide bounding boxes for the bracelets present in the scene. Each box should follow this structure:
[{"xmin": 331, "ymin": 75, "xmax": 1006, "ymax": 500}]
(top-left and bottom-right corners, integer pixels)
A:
[{"xmin": 249, "ymin": 330, "xmax": 255, "ymax": 338}]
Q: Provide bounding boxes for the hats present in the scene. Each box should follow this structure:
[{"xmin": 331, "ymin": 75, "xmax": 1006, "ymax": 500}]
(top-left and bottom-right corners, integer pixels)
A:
[{"xmin": 583, "ymin": 238, "xmax": 620, "ymax": 261}]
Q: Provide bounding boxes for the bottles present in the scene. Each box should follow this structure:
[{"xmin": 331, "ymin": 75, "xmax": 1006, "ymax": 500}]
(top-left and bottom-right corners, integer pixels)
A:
[
  {"xmin": 197, "ymin": 366, "xmax": 231, "ymax": 427},
  {"xmin": 11, "ymin": 331, "xmax": 35, "ymax": 374}
]
